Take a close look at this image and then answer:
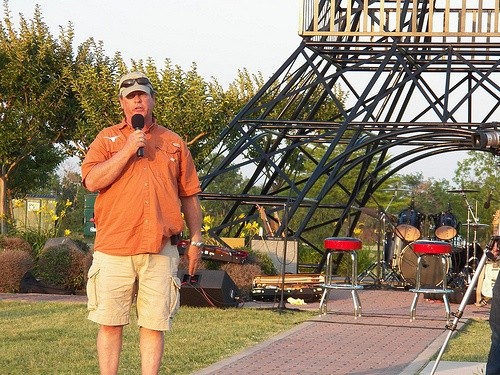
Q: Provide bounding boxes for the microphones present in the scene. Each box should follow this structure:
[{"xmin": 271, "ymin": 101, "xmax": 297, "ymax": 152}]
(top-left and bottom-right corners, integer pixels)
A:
[
  {"xmin": 484, "ymin": 194, "xmax": 492, "ymax": 209},
  {"xmin": 132, "ymin": 114, "xmax": 144, "ymax": 156}
]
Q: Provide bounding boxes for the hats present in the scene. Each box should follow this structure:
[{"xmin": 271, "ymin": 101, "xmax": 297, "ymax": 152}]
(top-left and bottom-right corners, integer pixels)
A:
[{"xmin": 119, "ymin": 71, "xmax": 153, "ymax": 99}]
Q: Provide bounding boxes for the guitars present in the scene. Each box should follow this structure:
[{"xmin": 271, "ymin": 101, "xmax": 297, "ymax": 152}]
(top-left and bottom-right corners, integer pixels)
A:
[{"xmin": 258, "ymin": 206, "xmax": 269, "ymax": 234}]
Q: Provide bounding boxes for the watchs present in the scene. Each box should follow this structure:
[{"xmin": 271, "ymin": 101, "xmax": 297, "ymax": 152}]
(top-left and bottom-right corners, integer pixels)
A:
[{"xmin": 190, "ymin": 241, "xmax": 204, "ymax": 249}]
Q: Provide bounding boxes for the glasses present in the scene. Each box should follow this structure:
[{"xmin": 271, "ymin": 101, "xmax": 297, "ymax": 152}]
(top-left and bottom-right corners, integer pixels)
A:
[{"xmin": 118, "ymin": 77, "xmax": 153, "ymax": 94}]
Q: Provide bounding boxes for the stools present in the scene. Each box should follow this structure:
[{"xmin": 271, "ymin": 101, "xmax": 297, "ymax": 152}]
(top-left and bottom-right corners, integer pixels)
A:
[
  {"xmin": 410, "ymin": 240, "xmax": 451, "ymax": 322},
  {"xmin": 319, "ymin": 237, "xmax": 362, "ymax": 319}
]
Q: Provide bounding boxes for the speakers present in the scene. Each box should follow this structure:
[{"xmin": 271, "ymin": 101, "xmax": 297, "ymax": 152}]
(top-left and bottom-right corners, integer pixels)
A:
[{"xmin": 178, "ymin": 270, "xmax": 241, "ymax": 307}]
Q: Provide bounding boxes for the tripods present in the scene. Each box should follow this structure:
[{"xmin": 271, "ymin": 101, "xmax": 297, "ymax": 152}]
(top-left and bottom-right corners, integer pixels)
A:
[{"xmin": 355, "ymin": 190, "xmax": 406, "ymax": 290}]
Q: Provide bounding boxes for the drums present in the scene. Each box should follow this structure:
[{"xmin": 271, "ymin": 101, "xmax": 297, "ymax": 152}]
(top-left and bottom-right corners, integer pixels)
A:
[
  {"xmin": 459, "ymin": 242, "xmax": 483, "ymax": 276},
  {"xmin": 383, "ymin": 231, "xmax": 406, "ymax": 268},
  {"xmin": 394, "ymin": 209, "xmax": 425, "ymax": 241},
  {"xmin": 434, "ymin": 211, "xmax": 459, "ymax": 239},
  {"xmin": 398, "ymin": 238, "xmax": 450, "ymax": 289}
]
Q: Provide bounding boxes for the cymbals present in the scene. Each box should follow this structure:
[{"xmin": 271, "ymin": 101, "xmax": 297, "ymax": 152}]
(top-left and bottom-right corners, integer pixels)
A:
[
  {"xmin": 360, "ymin": 207, "xmax": 398, "ymax": 223},
  {"xmin": 462, "ymin": 222, "xmax": 489, "ymax": 227}
]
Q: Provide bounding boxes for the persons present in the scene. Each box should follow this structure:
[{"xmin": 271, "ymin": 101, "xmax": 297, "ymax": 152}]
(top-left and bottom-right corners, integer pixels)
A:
[{"xmin": 81, "ymin": 71, "xmax": 204, "ymax": 375}]
